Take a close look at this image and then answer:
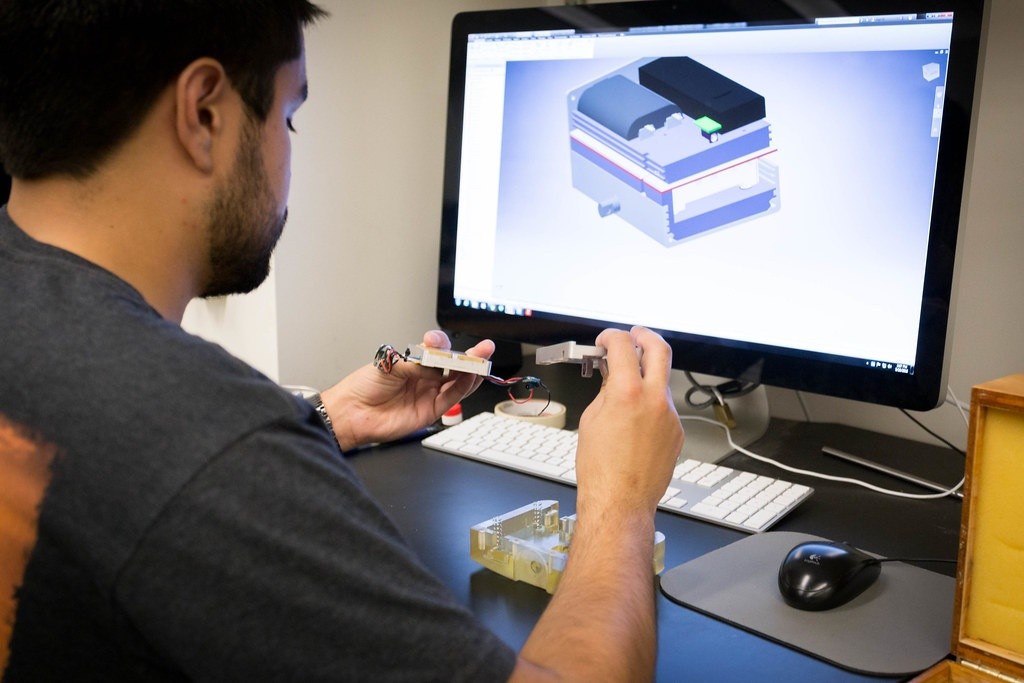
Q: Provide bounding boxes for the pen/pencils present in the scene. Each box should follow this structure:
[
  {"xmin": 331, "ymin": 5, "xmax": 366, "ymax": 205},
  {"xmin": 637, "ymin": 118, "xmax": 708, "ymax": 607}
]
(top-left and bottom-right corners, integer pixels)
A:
[{"xmin": 349, "ymin": 426, "xmax": 444, "ymax": 453}]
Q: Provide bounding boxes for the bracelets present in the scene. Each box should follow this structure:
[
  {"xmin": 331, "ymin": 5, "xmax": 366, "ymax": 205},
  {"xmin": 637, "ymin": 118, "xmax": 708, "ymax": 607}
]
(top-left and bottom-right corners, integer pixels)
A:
[{"xmin": 307, "ymin": 394, "xmax": 343, "ymax": 453}]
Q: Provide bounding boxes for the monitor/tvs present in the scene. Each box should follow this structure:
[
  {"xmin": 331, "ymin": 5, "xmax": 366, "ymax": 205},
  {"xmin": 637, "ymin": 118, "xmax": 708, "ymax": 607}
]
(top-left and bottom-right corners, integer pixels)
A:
[{"xmin": 435, "ymin": 0, "xmax": 984, "ymax": 411}]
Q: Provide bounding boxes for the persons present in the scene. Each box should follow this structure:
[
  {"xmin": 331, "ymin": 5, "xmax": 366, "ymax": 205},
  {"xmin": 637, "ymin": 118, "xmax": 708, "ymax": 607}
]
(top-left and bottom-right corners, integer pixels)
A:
[{"xmin": 0, "ymin": 1, "xmax": 685, "ymax": 682}]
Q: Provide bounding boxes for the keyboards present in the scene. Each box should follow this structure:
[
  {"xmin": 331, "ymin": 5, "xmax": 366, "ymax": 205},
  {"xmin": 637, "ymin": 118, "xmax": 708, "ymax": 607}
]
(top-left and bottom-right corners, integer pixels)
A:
[{"xmin": 421, "ymin": 411, "xmax": 815, "ymax": 534}]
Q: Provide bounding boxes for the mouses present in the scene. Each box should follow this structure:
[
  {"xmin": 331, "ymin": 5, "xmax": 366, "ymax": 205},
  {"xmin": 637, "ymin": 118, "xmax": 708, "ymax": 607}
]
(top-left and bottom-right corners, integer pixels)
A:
[{"xmin": 778, "ymin": 541, "xmax": 881, "ymax": 611}]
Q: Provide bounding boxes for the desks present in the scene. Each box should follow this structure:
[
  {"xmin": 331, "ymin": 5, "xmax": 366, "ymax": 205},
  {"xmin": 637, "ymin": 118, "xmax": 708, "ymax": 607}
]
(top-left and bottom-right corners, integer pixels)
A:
[{"xmin": 341, "ymin": 420, "xmax": 965, "ymax": 683}]
